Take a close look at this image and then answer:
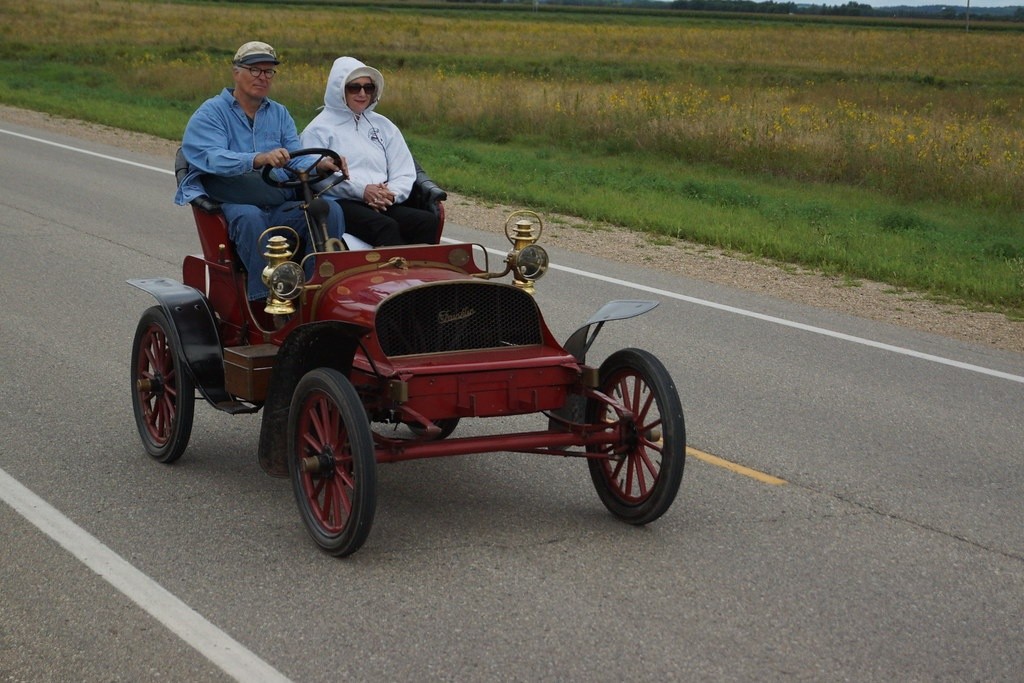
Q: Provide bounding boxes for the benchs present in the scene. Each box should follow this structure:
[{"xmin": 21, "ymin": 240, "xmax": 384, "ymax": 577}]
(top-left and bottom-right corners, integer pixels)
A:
[{"xmin": 174, "ymin": 146, "xmax": 448, "ymax": 263}]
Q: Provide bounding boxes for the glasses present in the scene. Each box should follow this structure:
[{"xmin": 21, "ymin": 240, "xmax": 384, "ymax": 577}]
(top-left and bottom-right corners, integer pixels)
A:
[
  {"xmin": 345, "ymin": 82, "xmax": 376, "ymax": 95},
  {"xmin": 237, "ymin": 64, "xmax": 276, "ymax": 79}
]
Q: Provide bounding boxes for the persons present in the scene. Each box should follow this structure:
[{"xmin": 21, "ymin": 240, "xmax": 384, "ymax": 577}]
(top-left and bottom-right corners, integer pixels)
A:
[
  {"xmin": 299, "ymin": 56, "xmax": 440, "ymax": 248},
  {"xmin": 173, "ymin": 41, "xmax": 350, "ymax": 301}
]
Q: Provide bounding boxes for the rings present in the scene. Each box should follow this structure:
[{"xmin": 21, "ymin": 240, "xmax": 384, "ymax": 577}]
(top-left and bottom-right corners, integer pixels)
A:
[{"xmin": 374, "ymin": 198, "xmax": 377, "ymax": 201}]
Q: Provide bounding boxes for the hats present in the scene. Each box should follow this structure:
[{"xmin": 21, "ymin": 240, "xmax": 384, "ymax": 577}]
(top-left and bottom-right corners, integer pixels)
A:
[
  {"xmin": 232, "ymin": 41, "xmax": 281, "ymax": 66},
  {"xmin": 345, "ymin": 68, "xmax": 377, "ymax": 86}
]
